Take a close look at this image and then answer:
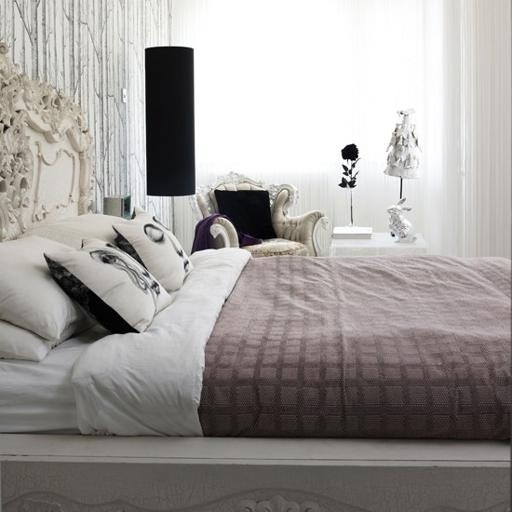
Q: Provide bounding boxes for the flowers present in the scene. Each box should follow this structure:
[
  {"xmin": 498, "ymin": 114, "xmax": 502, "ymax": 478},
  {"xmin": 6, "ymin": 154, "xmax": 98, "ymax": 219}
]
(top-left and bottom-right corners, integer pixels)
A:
[{"xmin": 338, "ymin": 144, "xmax": 360, "ymax": 226}]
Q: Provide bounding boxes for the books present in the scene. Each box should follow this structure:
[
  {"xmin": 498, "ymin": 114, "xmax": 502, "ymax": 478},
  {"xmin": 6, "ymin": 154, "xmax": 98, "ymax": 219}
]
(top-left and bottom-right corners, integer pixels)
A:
[{"xmin": 332, "ymin": 227, "xmax": 373, "ymax": 239}]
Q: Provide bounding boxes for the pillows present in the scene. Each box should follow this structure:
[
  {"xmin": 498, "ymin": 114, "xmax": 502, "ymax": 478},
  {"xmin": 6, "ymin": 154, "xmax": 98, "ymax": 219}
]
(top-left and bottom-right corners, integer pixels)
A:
[
  {"xmin": 214, "ymin": 186, "xmax": 276, "ymax": 239},
  {"xmin": 0, "ymin": 196, "xmax": 192, "ymax": 363}
]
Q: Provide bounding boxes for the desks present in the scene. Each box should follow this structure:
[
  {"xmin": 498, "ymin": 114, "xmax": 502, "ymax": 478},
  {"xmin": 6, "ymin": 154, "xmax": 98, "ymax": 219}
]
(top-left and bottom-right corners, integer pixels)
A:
[{"xmin": 332, "ymin": 234, "xmax": 421, "ymax": 256}]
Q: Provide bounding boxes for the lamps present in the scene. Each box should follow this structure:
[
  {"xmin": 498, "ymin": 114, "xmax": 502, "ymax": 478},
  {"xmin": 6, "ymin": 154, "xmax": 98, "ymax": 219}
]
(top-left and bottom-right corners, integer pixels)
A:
[{"xmin": 145, "ymin": 46, "xmax": 196, "ymax": 238}]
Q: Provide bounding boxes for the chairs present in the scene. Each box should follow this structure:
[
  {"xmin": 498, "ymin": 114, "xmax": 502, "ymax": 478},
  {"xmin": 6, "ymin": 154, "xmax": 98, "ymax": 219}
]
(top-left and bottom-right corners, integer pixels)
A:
[{"xmin": 198, "ymin": 173, "xmax": 328, "ymax": 257}]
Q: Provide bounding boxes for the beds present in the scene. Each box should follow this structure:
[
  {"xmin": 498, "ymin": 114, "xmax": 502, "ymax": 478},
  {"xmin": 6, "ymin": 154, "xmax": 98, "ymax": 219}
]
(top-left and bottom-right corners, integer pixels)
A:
[{"xmin": 0, "ymin": 63, "xmax": 511, "ymax": 512}]
